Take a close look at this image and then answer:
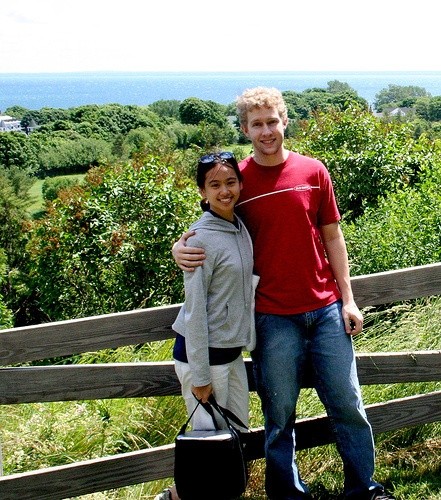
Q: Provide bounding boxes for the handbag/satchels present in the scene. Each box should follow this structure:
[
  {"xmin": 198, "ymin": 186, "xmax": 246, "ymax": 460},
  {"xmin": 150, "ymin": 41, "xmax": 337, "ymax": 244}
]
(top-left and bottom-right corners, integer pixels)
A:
[{"xmin": 173, "ymin": 392, "xmax": 247, "ymax": 500}]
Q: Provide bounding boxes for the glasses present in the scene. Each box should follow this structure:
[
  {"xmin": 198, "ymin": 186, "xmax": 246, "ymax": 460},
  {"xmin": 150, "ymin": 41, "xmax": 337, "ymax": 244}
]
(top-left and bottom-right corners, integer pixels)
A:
[{"xmin": 198, "ymin": 151, "xmax": 232, "ymax": 164}]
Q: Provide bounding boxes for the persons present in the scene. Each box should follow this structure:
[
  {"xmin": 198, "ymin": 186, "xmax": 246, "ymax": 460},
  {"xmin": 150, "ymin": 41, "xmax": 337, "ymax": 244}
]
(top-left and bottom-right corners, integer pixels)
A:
[
  {"xmin": 153, "ymin": 151, "xmax": 257, "ymax": 500},
  {"xmin": 171, "ymin": 84, "xmax": 392, "ymax": 499}
]
